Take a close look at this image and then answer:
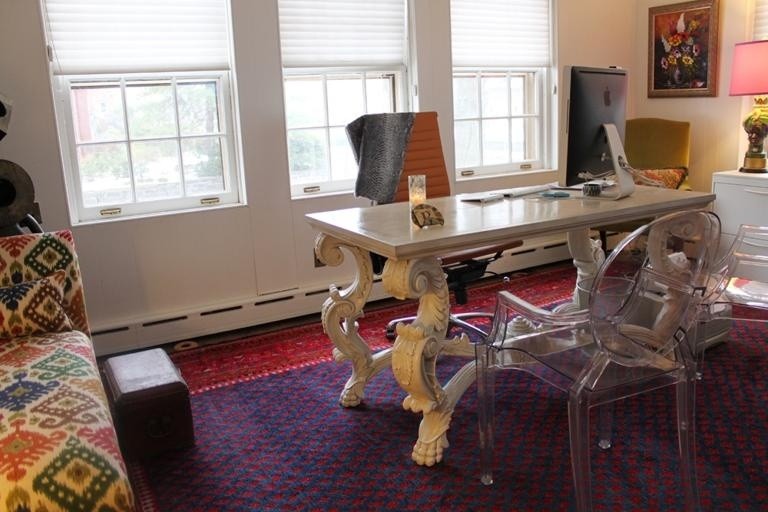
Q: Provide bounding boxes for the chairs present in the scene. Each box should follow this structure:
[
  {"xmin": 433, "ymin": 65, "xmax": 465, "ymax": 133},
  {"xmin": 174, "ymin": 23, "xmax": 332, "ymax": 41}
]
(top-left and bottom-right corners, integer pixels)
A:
[
  {"xmin": 587, "ymin": 117, "xmax": 692, "ymax": 252},
  {"xmin": 474, "ymin": 210, "xmax": 719, "ymax": 509},
  {"xmin": 357, "ymin": 110, "xmax": 522, "ymax": 344}
]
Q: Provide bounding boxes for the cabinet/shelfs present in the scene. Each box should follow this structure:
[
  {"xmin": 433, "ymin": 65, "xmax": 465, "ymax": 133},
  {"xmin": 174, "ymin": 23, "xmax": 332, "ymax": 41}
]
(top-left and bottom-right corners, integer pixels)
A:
[{"xmin": 710, "ymin": 169, "xmax": 768, "ymax": 284}]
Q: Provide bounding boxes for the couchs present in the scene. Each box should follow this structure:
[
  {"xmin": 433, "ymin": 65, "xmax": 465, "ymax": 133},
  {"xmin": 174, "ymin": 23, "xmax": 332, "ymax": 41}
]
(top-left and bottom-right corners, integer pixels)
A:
[{"xmin": 1, "ymin": 229, "xmax": 138, "ymax": 512}]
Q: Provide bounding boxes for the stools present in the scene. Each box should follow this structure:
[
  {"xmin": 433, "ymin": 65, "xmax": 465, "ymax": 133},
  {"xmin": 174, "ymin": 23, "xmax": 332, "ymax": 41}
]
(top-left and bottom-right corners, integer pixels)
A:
[{"xmin": 103, "ymin": 349, "xmax": 196, "ymax": 462}]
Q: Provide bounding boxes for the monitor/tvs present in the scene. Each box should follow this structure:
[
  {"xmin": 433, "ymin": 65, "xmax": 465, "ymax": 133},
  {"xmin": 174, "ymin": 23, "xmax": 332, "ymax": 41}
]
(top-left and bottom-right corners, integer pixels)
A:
[{"xmin": 559, "ymin": 63, "xmax": 636, "ymax": 201}]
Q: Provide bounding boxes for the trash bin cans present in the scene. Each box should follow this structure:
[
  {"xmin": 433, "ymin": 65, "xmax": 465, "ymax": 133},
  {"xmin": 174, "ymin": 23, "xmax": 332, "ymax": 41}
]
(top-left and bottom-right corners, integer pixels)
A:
[{"xmin": 576, "ymin": 277, "xmax": 636, "ymax": 358}]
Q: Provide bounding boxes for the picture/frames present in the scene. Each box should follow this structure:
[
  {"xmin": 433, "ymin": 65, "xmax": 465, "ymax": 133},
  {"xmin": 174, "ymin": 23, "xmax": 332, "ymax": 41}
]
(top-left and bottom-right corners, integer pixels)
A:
[{"xmin": 647, "ymin": 1, "xmax": 720, "ymax": 98}]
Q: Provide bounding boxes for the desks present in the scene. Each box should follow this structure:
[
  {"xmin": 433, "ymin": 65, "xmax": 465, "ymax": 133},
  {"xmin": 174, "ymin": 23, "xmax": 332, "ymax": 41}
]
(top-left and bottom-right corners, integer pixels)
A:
[{"xmin": 306, "ymin": 185, "xmax": 715, "ymax": 472}]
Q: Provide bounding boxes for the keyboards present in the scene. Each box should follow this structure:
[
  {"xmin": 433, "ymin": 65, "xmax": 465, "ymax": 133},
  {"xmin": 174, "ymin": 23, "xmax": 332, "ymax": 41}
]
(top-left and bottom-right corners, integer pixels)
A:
[{"xmin": 461, "ymin": 185, "xmax": 549, "ymax": 204}]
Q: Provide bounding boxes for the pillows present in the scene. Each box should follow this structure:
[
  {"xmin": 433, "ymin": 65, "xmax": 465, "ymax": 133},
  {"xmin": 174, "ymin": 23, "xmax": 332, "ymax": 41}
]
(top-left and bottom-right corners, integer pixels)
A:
[{"xmin": 632, "ymin": 167, "xmax": 687, "ymax": 190}]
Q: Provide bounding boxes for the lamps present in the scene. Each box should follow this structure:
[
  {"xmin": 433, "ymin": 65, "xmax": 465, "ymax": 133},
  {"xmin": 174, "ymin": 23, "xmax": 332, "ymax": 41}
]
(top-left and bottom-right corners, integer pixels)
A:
[{"xmin": 728, "ymin": 39, "xmax": 768, "ymax": 173}]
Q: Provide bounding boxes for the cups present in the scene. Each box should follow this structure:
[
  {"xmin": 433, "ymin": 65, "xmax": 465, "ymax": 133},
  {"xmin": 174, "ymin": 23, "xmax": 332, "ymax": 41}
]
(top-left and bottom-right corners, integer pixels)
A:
[{"xmin": 408, "ymin": 175, "xmax": 426, "ymax": 208}]
